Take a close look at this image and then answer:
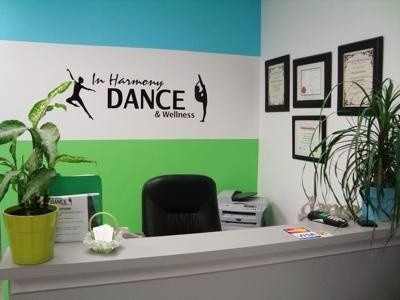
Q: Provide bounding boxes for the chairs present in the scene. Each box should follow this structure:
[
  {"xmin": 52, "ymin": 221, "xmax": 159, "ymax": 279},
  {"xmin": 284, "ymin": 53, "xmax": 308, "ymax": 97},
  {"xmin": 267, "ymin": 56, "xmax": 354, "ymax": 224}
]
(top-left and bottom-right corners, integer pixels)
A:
[{"xmin": 142, "ymin": 175, "xmax": 222, "ymax": 237}]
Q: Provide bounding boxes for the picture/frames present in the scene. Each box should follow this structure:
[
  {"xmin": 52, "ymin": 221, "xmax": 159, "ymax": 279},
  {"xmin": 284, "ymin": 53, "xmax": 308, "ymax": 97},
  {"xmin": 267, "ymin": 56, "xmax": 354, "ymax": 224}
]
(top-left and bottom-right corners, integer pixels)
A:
[{"xmin": 265, "ymin": 37, "xmax": 383, "ymax": 163}]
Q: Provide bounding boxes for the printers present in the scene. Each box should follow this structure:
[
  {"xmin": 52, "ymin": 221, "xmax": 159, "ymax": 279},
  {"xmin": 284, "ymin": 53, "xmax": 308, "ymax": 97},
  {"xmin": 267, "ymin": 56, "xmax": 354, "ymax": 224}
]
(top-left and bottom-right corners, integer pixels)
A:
[{"xmin": 218, "ymin": 189, "xmax": 271, "ymax": 230}]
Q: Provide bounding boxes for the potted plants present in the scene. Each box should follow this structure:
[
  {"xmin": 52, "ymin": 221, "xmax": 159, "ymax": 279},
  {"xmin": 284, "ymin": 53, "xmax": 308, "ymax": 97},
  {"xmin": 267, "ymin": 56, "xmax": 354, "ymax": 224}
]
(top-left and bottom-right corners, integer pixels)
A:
[
  {"xmin": 0, "ymin": 80, "xmax": 96, "ymax": 265},
  {"xmin": 302, "ymin": 80, "xmax": 400, "ymax": 252}
]
